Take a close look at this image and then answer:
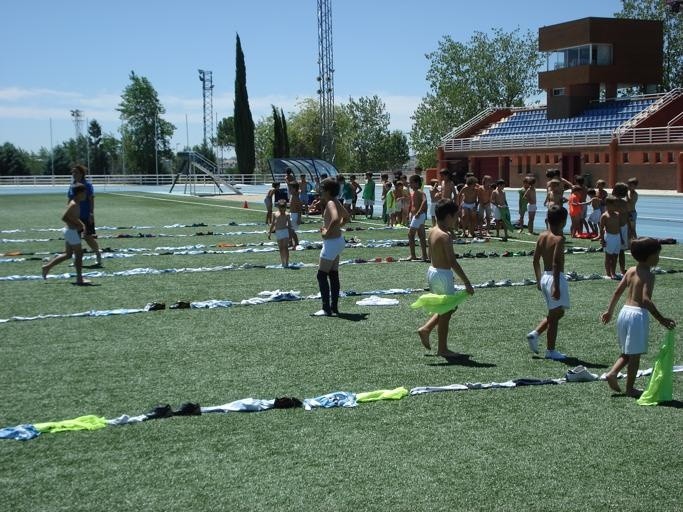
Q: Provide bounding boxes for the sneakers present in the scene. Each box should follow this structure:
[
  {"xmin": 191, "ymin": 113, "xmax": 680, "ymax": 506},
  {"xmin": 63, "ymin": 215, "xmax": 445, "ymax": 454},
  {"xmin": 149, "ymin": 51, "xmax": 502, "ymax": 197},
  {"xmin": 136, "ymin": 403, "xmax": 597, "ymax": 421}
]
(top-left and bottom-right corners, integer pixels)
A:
[
  {"xmin": 463, "ymin": 250, "xmax": 475, "ymax": 258},
  {"xmin": 452, "ymin": 239, "xmax": 466, "ymax": 244},
  {"xmin": 169, "ymin": 301, "xmax": 190, "ymax": 309},
  {"xmin": 652, "ymin": 269, "xmax": 666, "ymax": 274},
  {"xmin": 481, "ymin": 280, "xmax": 495, "ymax": 287},
  {"xmin": 267, "ymin": 290, "xmax": 284, "ymax": 302},
  {"xmin": 576, "ymin": 274, "xmax": 589, "ymax": 279},
  {"xmin": 281, "ymin": 293, "xmax": 298, "ymax": 300},
  {"xmin": 355, "ymin": 259, "xmax": 368, "ymax": 263},
  {"xmin": 490, "ymin": 253, "xmax": 499, "ymax": 257},
  {"xmin": 275, "ymin": 397, "xmax": 302, "ymax": 408},
  {"xmin": 590, "ymin": 273, "xmax": 605, "ymax": 280},
  {"xmin": 368, "ymin": 257, "xmax": 382, "ymax": 263},
  {"xmin": 145, "ymin": 405, "xmax": 172, "ymax": 419},
  {"xmin": 385, "ymin": 257, "xmax": 394, "ymax": 262},
  {"xmin": 172, "ymin": 402, "xmax": 201, "ymax": 415},
  {"xmin": 495, "ymin": 279, "xmax": 512, "ymax": 286},
  {"xmin": 513, "ymin": 250, "xmax": 525, "ymax": 256},
  {"xmin": 564, "ymin": 271, "xmax": 576, "ymax": 282},
  {"xmin": 145, "ymin": 302, "xmax": 165, "ymax": 310},
  {"xmin": 476, "ymin": 250, "xmax": 488, "ymax": 258},
  {"xmin": 502, "ymin": 251, "xmax": 513, "ymax": 256},
  {"xmin": 473, "ymin": 238, "xmax": 486, "ymax": 243},
  {"xmin": 565, "ymin": 365, "xmax": 598, "ymax": 382},
  {"xmin": 523, "ymin": 279, "xmax": 537, "ymax": 284}
]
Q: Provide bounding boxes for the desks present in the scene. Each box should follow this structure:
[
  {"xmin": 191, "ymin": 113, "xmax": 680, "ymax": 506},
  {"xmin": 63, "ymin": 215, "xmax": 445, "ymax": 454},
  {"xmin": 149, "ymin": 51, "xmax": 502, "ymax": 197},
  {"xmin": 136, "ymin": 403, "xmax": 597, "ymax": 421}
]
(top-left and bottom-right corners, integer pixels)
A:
[{"xmin": 275, "ymin": 188, "xmax": 319, "ymax": 203}]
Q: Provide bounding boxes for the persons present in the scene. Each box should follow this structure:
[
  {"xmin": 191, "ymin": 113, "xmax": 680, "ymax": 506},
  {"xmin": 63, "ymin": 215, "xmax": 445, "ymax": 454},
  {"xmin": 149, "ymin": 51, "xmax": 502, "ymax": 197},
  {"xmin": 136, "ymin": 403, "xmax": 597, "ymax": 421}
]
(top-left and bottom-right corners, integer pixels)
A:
[
  {"xmin": 66, "ymin": 165, "xmax": 102, "ymax": 265},
  {"xmin": 310, "ymin": 176, "xmax": 351, "ymax": 317},
  {"xmin": 42, "ymin": 183, "xmax": 86, "ymax": 285},
  {"xmin": 526, "ymin": 204, "xmax": 570, "ymax": 361},
  {"xmin": 417, "ymin": 198, "xmax": 474, "ymax": 357},
  {"xmin": 602, "ymin": 235, "xmax": 676, "ymax": 396},
  {"xmin": 263, "ymin": 167, "xmax": 638, "ymax": 280}
]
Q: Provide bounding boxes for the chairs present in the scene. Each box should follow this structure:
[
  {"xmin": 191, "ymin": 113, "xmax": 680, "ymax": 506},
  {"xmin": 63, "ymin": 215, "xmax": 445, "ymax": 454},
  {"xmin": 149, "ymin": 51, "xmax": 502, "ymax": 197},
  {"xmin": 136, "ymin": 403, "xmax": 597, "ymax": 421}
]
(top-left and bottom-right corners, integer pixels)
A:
[{"xmin": 471, "ymin": 100, "xmax": 657, "ymax": 141}]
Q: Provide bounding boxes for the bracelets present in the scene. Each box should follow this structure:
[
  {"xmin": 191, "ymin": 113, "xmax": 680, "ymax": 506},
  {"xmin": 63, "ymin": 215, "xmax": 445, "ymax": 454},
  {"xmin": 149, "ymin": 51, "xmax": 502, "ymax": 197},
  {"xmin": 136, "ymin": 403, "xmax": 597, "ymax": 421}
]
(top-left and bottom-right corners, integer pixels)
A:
[{"xmin": 90, "ymin": 212, "xmax": 94, "ymax": 216}]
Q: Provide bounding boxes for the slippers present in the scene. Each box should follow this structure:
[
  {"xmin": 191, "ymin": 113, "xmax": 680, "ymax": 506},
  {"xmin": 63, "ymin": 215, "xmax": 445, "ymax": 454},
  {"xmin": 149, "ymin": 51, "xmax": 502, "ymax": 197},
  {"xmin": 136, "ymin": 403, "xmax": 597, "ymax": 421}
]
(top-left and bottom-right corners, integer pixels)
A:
[{"xmin": 345, "ymin": 290, "xmax": 361, "ymax": 296}]
